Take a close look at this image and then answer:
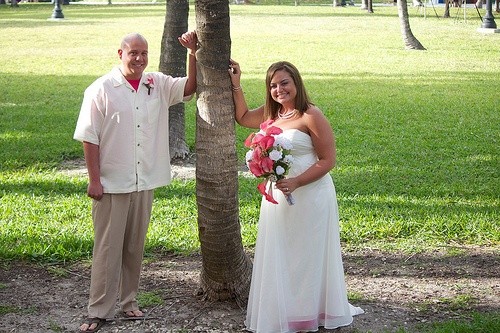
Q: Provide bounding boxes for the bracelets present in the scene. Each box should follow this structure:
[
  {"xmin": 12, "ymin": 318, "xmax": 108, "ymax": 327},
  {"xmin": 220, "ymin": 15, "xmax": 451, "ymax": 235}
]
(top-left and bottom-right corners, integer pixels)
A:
[
  {"xmin": 189, "ymin": 53, "xmax": 195, "ymax": 57},
  {"xmin": 232, "ymin": 86, "xmax": 241, "ymax": 91}
]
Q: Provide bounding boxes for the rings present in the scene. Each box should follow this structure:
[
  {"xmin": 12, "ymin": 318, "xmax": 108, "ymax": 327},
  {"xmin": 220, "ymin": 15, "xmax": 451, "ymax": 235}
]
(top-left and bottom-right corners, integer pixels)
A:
[{"xmin": 286, "ymin": 188, "xmax": 288, "ymax": 191}]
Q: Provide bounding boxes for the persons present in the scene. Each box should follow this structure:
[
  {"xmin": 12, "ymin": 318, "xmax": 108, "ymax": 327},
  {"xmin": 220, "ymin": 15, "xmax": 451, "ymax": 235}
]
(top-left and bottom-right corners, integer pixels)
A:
[
  {"xmin": 229, "ymin": 59, "xmax": 364, "ymax": 333},
  {"xmin": 73, "ymin": 28, "xmax": 197, "ymax": 333}
]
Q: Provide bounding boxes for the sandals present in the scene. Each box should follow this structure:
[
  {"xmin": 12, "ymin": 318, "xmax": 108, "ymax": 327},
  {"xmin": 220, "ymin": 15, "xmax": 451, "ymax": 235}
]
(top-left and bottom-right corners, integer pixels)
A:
[
  {"xmin": 121, "ymin": 310, "xmax": 144, "ymax": 319},
  {"xmin": 78, "ymin": 318, "xmax": 106, "ymax": 333}
]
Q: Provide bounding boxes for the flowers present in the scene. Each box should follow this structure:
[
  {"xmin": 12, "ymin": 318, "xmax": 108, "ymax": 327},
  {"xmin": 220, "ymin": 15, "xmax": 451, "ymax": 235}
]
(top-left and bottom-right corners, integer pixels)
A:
[
  {"xmin": 245, "ymin": 120, "xmax": 296, "ymax": 206},
  {"xmin": 143, "ymin": 78, "xmax": 155, "ymax": 95}
]
